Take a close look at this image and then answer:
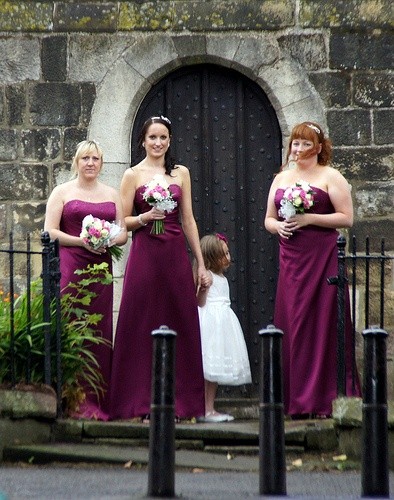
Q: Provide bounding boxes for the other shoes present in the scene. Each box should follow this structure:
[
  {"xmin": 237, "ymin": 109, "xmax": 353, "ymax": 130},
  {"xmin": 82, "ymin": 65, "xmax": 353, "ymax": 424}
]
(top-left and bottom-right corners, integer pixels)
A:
[
  {"xmin": 197, "ymin": 412, "xmax": 228, "ymax": 422},
  {"xmin": 213, "ymin": 410, "xmax": 235, "ymax": 421}
]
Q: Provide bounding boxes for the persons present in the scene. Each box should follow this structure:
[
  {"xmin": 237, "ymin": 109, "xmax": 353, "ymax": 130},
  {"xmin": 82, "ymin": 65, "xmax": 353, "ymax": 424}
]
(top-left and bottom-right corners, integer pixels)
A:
[
  {"xmin": 264, "ymin": 122, "xmax": 362, "ymax": 419},
  {"xmin": 120, "ymin": 116, "xmax": 207, "ymax": 423},
  {"xmin": 193, "ymin": 233, "xmax": 252, "ymax": 422},
  {"xmin": 44, "ymin": 141, "xmax": 128, "ymax": 421}
]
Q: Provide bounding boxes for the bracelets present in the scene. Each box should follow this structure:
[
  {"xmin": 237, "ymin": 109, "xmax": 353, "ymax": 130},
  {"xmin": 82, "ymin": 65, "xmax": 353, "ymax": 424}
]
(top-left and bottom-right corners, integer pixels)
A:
[{"xmin": 137, "ymin": 213, "xmax": 148, "ymax": 227}]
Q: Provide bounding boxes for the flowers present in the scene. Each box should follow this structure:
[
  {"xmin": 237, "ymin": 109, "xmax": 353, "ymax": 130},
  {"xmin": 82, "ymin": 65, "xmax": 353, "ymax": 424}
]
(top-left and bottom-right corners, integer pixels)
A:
[
  {"xmin": 79, "ymin": 214, "xmax": 126, "ymax": 264},
  {"xmin": 140, "ymin": 174, "xmax": 177, "ymax": 235},
  {"xmin": 278, "ymin": 180, "xmax": 316, "ymax": 219}
]
